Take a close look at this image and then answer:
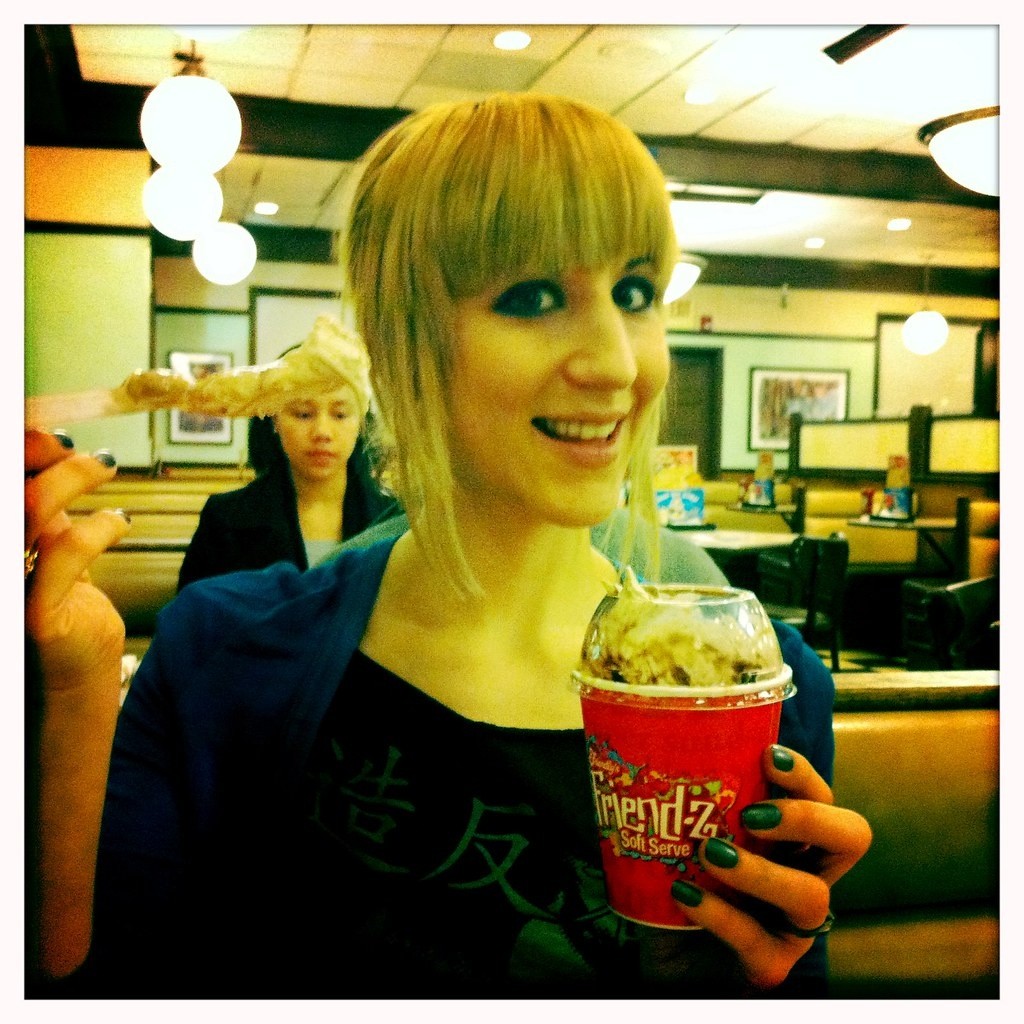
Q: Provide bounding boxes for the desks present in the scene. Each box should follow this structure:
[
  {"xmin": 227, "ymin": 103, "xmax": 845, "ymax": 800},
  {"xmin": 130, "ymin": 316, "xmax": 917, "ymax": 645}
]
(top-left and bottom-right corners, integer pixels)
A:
[
  {"xmin": 729, "ymin": 505, "xmax": 797, "ymax": 529},
  {"xmin": 680, "ymin": 528, "xmax": 800, "ymax": 554},
  {"xmin": 849, "ymin": 519, "xmax": 956, "ymax": 573}
]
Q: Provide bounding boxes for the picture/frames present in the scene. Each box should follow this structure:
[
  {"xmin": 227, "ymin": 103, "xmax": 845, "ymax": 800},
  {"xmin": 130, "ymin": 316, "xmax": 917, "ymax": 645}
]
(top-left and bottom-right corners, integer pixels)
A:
[
  {"xmin": 748, "ymin": 367, "xmax": 851, "ymax": 453},
  {"xmin": 165, "ymin": 348, "xmax": 234, "ymax": 445}
]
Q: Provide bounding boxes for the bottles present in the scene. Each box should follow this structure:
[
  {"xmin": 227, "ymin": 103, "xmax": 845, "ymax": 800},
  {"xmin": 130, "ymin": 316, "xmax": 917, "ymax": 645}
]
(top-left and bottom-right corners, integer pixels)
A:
[
  {"xmin": 752, "ymin": 455, "xmax": 773, "ymax": 506},
  {"xmin": 886, "ymin": 456, "xmax": 911, "ymax": 520},
  {"xmin": 651, "ymin": 446, "xmax": 704, "ymax": 527}
]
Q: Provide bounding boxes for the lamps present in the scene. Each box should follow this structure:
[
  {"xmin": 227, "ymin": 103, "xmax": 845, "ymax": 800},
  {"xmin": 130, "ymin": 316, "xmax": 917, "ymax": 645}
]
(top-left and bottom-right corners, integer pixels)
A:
[
  {"xmin": 919, "ymin": 106, "xmax": 1000, "ymax": 196},
  {"xmin": 139, "ymin": 39, "xmax": 258, "ymax": 285},
  {"xmin": 902, "ymin": 253, "xmax": 949, "ymax": 355},
  {"xmin": 663, "ymin": 252, "xmax": 709, "ymax": 305}
]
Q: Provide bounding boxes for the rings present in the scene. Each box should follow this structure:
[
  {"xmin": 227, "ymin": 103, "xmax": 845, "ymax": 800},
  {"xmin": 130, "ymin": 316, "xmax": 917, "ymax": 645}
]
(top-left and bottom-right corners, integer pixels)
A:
[
  {"xmin": 24, "ymin": 547, "xmax": 39, "ymax": 582},
  {"xmin": 787, "ymin": 908, "xmax": 835, "ymax": 938}
]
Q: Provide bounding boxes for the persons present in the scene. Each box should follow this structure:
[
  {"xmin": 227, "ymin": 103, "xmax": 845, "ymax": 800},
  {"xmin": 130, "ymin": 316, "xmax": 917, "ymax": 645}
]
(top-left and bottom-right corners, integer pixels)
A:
[
  {"xmin": 176, "ymin": 345, "xmax": 406, "ymax": 593},
  {"xmin": 24, "ymin": 91, "xmax": 873, "ymax": 1001}
]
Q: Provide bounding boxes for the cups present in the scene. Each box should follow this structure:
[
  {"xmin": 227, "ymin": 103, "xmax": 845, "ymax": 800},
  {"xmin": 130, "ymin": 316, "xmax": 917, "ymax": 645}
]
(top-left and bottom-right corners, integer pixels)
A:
[{"xmin": 567, "ymin": 583, "xmax": 798, "ymax": 930}]
